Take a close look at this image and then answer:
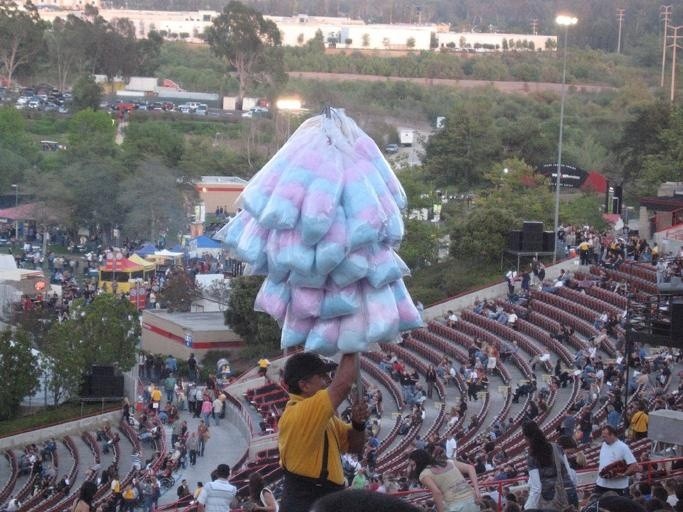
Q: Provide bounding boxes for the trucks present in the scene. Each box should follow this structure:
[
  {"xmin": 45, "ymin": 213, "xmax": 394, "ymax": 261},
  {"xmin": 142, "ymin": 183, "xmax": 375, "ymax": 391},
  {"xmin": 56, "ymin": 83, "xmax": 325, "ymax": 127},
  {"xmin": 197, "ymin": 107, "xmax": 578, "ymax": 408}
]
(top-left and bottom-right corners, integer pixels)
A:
[{"xmin": 398, "ymin": 126, "xmax": 413, "ymax": 146}]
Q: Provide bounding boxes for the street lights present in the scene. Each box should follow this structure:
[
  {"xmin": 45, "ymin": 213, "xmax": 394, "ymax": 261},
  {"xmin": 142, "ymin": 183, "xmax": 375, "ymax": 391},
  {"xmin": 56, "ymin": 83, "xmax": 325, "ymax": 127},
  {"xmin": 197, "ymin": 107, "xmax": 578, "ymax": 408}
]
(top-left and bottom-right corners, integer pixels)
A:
[
  {"xmin": 625, "ymin": 206, "xmax": 635, "ymax": 225},
  {"xmin": 111, "ymin": 246, "xmax": 122, "ymax": 297},
  {"xmin": 552, "ymin": 12, "xmax": 580, "ymax": 267},
  {"xmin": 10, "ymin": 184, "xmax": 18, "ymax": 241}
]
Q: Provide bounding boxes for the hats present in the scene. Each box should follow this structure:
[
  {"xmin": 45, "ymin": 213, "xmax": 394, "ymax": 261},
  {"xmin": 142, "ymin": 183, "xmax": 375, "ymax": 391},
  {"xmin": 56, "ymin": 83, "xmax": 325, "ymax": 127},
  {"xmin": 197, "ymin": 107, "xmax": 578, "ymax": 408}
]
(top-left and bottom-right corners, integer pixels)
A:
[{"xmin": 282, "ymin": 350, "xmax": 338, "ymax": 387}]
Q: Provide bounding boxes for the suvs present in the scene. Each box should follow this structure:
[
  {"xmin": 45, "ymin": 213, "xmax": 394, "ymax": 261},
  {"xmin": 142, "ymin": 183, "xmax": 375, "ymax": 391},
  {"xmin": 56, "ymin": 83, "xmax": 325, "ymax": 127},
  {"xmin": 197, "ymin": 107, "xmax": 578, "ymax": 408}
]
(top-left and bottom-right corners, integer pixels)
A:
[{"xmin": 385, "ymin": 142, "xmax": 399, "ymax": 154}]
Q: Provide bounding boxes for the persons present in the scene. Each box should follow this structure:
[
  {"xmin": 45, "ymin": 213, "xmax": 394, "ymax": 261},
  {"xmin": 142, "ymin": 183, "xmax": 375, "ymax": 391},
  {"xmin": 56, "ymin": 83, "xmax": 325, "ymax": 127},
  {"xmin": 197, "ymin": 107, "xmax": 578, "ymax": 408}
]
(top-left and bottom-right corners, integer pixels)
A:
[
  {"xmin": 0, "ymin": 204, "xmax": 246, "ymax": 324},
  {"xmin": 340, "ymin": 337, "xmax": 683, "ymax": 511},
  {"xmin": 1, "ymin": 422, "xmax": 284, "ymax": 512},
  {"xmin": 120, "ymin": 349, "xmax": 275, "ymax": 437},
  {"xmin": 557, "ymin": 223, "xmax": 659, "ymax": 266},
  {"xmin": 416, "ymin": 257, "xmax": 682, "ymax": 350}
]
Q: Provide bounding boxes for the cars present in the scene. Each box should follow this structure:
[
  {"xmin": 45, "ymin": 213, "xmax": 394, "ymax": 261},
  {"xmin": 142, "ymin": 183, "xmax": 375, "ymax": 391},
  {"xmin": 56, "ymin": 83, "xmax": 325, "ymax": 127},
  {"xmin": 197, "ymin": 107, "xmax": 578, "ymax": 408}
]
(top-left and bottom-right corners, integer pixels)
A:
[
  {"xmin": 0, "ymin": 81, "xmax": 72, "ymax": 116},
  {"xmin": 98, "ymin": 97, "xmax": 268, "ymax": 122}
]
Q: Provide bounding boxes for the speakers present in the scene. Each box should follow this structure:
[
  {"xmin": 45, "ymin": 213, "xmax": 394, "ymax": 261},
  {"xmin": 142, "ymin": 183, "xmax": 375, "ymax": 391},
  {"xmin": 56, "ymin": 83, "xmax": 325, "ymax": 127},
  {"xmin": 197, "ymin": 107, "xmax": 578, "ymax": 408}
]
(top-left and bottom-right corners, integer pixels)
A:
[
  {"xmin": 80, "ymin": 364, "xmax": 124, "ymax": 399},
  {"xmin": 510, "ymin": 221, "xmax": 555, "ymax": 252},
  {"xmin": 653, "ymin": 295, "xmax": 683, "ymax": 337}
]
